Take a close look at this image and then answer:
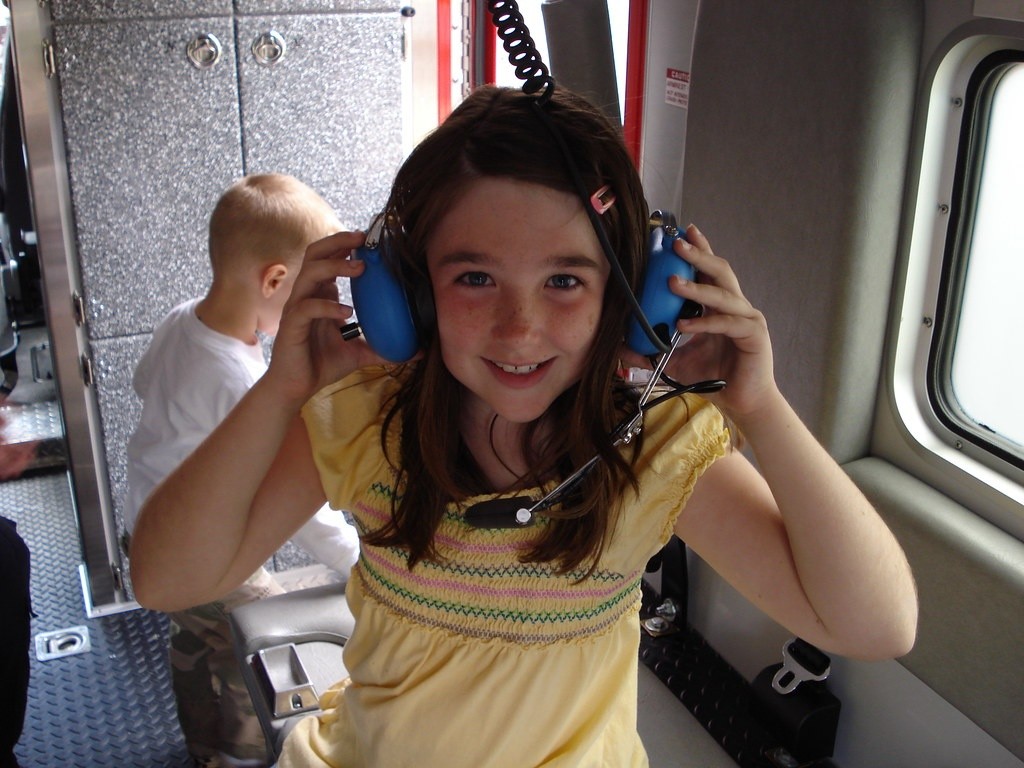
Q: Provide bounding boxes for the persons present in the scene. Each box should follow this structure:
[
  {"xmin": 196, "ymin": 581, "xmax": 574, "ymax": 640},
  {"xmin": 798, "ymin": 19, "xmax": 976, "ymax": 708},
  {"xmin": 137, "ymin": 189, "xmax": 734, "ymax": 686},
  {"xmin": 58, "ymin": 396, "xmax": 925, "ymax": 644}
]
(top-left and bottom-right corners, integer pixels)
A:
[
  {"xmin": 125, "ymin": 169, "xmax": 360, "ymax": 768},
  {"xmin": 128, "ymin": 77, "xmax": 917, "ymax": 768}
]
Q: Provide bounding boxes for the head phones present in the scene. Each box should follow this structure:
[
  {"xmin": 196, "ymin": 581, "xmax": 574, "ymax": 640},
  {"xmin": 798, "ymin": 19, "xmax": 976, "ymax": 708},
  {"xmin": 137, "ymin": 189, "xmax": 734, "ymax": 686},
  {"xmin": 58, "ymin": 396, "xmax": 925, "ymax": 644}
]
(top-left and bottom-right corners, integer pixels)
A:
[{"xmin": 351, "ymin": 213, "xmax": 704, "ymax": 365}]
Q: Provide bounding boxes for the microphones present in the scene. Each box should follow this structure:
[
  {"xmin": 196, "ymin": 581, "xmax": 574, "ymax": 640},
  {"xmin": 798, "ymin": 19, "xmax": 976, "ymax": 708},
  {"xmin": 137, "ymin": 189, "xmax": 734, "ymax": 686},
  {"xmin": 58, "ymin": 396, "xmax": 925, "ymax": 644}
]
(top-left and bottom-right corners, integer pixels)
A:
[{"xmin": 465, "ymin": 330, "xmax": 684, "ymax": 526}]
